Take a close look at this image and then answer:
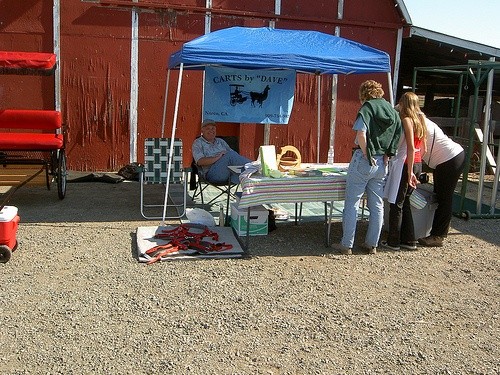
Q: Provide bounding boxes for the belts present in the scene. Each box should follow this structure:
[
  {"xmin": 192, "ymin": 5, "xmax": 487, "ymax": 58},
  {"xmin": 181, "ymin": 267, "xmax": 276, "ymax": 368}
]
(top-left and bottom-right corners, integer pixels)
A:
[{"xmin": 357, "ymin": 145, "xmax": 361, "ymax": 149}]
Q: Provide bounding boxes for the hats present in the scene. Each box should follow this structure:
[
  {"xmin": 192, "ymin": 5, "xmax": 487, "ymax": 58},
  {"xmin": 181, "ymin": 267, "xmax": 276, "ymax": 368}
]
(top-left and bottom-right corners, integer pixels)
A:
[{"xmin": 202, "ymin": 119, "xmax": 216, "ymax": 127}]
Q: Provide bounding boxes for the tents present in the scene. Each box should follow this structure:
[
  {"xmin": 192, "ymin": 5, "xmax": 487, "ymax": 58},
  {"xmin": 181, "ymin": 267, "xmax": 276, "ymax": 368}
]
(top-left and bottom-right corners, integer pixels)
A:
[{"xmin": 161, "ymin": 26, "xmax": 395, "ymax": 227}]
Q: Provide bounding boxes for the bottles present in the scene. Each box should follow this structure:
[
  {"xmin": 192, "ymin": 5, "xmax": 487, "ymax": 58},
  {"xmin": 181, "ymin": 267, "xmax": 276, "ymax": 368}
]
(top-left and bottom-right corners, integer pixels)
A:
[{"xmin": 328, "ymin": 146, "xmax": 334, "ymax": 165}]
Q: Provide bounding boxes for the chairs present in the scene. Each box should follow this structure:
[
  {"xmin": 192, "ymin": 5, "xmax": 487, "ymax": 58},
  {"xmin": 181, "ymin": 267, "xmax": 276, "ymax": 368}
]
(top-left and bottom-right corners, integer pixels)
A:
[
  {"xmin": 139, "ymin": 136, "xmax": 187, "ymax": 220},
  {"xmin": 189, "ymin": 135, "xmax": 242, "ymax": 212}
]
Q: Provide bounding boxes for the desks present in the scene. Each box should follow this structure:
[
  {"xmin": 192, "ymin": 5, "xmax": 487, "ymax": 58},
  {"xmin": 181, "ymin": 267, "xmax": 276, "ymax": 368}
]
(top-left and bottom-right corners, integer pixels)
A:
[{"xmin": 226, "ymin": 163, "xmax": 367, "ymax": 259}]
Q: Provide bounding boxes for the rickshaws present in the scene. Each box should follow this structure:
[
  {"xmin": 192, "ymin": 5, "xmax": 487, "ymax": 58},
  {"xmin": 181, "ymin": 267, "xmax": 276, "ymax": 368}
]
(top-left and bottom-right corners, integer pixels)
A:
[{"xmin": 0, "ymin": 50, "xmax": 68, "ymax": 211}]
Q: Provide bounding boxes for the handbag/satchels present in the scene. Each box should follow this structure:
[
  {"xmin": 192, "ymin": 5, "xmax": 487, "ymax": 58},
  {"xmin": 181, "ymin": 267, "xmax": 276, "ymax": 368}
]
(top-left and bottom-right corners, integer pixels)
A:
[{"xmin": 419, "ymin": 173, "xmax": 429, "ymax": 183}]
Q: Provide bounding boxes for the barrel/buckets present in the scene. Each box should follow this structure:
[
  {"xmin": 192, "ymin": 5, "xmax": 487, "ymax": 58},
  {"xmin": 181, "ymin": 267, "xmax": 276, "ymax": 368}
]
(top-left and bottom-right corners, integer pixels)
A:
[{"xmin": 384, "ymin": 183, "xmax": 438, "ymax": 240}]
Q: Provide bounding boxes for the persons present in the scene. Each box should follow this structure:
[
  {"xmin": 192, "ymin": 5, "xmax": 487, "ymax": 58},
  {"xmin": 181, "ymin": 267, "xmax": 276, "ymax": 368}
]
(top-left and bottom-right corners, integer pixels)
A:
[
  {"xmin": 381, "ymin": 92, "xmax": 428, "ymax": 250},
  {"xmin": 332, "ymin": 80, "xmax": 402, "ymax": 254},
  {"xmin": 191, "ymin": 119, "xmax": 254, "ymax": 184},
  {"xmin": 394, "ymin": 103, "xmax": 465, "ymax": 246}
]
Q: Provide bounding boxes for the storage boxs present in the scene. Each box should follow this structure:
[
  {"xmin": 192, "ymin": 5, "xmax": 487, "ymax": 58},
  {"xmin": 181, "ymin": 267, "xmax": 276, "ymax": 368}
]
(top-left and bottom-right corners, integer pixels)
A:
[
  {"xmin": 230, "ymin": 203, "xmax": 269, "ymax": 237},
  {"xmin": 0, "ymin": 206, "xmax": 20, "ymax": 250}
]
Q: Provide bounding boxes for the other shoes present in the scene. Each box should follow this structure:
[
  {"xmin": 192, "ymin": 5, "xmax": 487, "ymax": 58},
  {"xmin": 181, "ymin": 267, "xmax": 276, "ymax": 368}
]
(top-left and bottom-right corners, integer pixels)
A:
[
  {"xmin": 400, "ymin": 241, "xmax": 417, "ymax": 250},
  {"xmin": 360, "ymin": 242, "xmax": 377, "ymax": 254},
  {"xmin": 381, "ymin": 240, "xmax": 400, "ymax": 250},
  {"xmin": 331, "ymin": 243, "xmax": 352, "ymax": 255},
  {"xmin": 417, "ymin": 234, "xmax": 444, "ymax": 247}
]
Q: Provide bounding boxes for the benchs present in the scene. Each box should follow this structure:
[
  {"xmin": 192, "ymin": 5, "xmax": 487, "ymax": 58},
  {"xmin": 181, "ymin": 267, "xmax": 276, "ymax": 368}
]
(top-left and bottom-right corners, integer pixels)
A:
[{"xmin": 0, "ymin": 110, "xmax": 64, "ymax": 151}]
